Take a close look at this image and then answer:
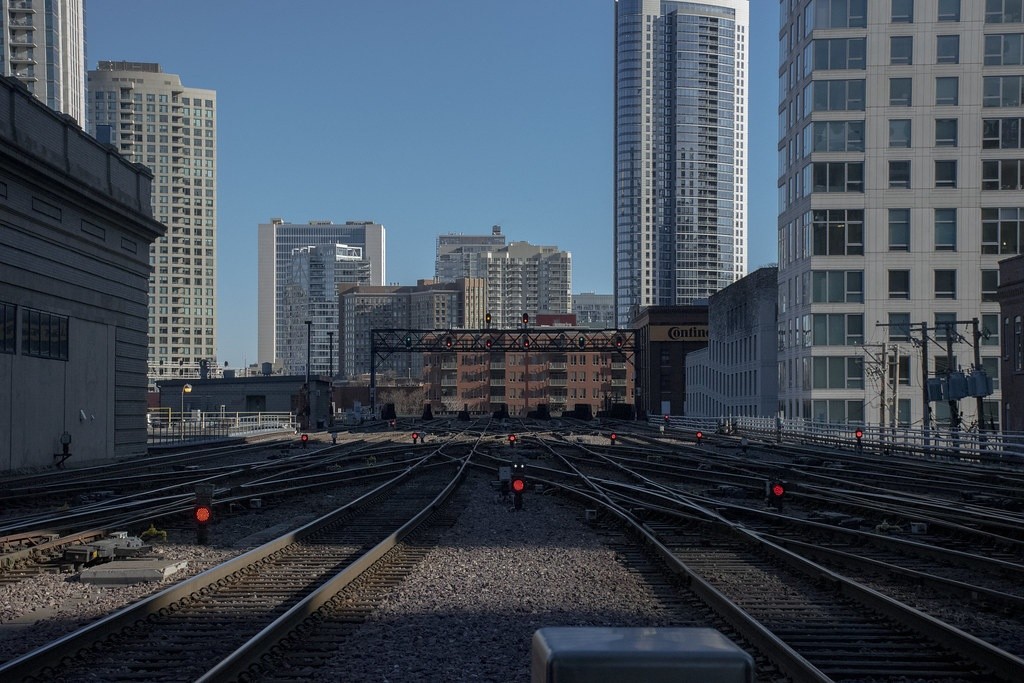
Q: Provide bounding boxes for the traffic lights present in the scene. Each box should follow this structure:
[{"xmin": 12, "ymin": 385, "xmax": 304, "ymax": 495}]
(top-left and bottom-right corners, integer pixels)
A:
[
  {"xmin": 302, "ymin": 434, "xmax": 308, "ymax": 443},
  {"xmin": 487, "ymin": 340, "xmax": 492, "ymax": 347},
  {"xmin": 696, "ymin": 431, "xmax": 702, "ymax": 438},
  {"xmin": 769, "ymin": 483, "xmax": 784, "ymax": 512},
  {"xmin": 412, "ymin": 433, "xmax": 418, "ymax": 439},
  {"xmin": 524, "ymin": 339, "xmax": 529, "ymax": 347},
  {"xmin": 446, "ymin": 337, "xmax": 452, "ymax": 347},
  {"xmin": 508, "ymin": 435, "xmax": 516, "ymax": 442},
  {"xmin": 664, "ymin": 415, "xmax": 669, "ymax": 419},
  {"xmin": 523, "ymin": 313, "xmax": 528, "ymax": 323},
  {"xmin": 855, "ymin": 428, "xmax": 862, "ymax": 438},
  {"xmin": 196, "ymin": 484, "xmax": 213, "ymax": 526},
  {"xmin": 406, "ymin": 337, "xmax": 411, "ymax": 347},
  {"xmin": 616, "ymin": 336, "xmax": 622, "ymax": 347},
  {"xmin": 511, "ymin": 462, "xmax": 526, "ymax": 494},
  {"xmin": 610, "ymin": 432, "xmax": 617, "ymax": 440},
  {"xmin": 486, "ymin": 314, "xmax": 491, "ymax": 323},
  {"xmin": 579, "ymin": 337, "xmax": 584, "ymax": 347}
]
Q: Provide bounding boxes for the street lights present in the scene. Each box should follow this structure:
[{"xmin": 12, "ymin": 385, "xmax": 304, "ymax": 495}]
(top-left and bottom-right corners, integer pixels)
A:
[{"xmin": 181, "ymin": 383, "xmax": 193, "ymax": 441}]
